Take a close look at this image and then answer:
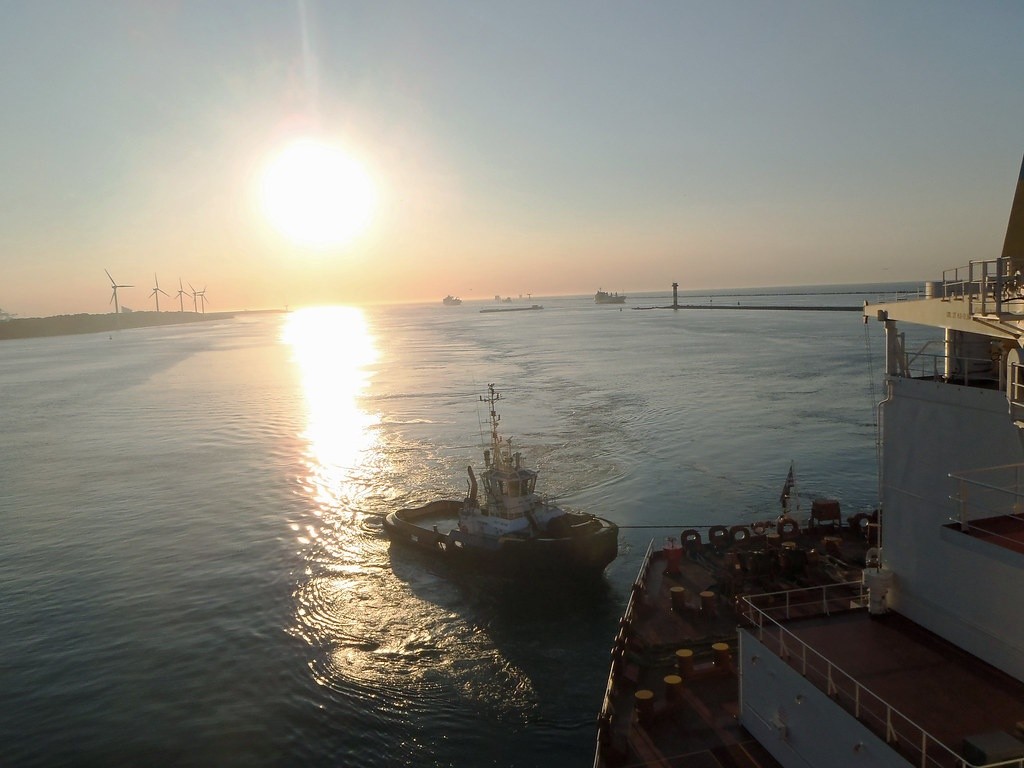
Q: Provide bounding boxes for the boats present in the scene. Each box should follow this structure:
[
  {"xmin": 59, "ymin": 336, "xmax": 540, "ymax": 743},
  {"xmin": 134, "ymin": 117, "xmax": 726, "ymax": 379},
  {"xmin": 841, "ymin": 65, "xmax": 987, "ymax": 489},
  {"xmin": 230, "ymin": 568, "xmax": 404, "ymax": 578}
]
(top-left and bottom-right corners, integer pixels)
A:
[
  {"xmin": 600, "ymin": 153, "xmax": 1024, "ymax": 768},
  {"xmin": 381, "ymin": 381, "xmax": 621, "ymax": 597},
  {"xmin": 443, "ymin": 295, "xmax": 463, "ymax": 306},
  {"xmin": 595, "ymin": 290, "xmax": 626, "ymax": 304}
]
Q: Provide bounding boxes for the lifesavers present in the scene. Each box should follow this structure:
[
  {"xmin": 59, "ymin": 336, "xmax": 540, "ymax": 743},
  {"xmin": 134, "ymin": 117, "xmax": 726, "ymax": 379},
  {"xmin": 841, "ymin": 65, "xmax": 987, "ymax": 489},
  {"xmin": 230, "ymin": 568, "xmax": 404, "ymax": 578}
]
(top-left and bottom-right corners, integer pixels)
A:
[
  {"xmin": 776, "ymin": 519, "xmax": 799, "ymax": 536},
  {"xmin": 850, "ymin": 511, "xmax": 871, "ymax": 533},
  {"xmin": 728, "ymin": 525, "xmax": 752, "ymax": 543},
  {"xmin": 681, "ymin": 528, "xmax": 703, "ymax": 549},
  {"xmin": 708, "ymin": 525, "xmax": 727, "ymax": 542}
]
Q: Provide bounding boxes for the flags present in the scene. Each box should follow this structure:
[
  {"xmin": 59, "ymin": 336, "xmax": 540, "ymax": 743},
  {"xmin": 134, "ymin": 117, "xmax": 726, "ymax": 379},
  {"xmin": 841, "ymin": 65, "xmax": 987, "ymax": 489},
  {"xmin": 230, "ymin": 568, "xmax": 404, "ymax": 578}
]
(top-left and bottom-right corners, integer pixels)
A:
[{"xmin": 780, "ymin": 466, "xmax": 793, "ymax": 509}]
